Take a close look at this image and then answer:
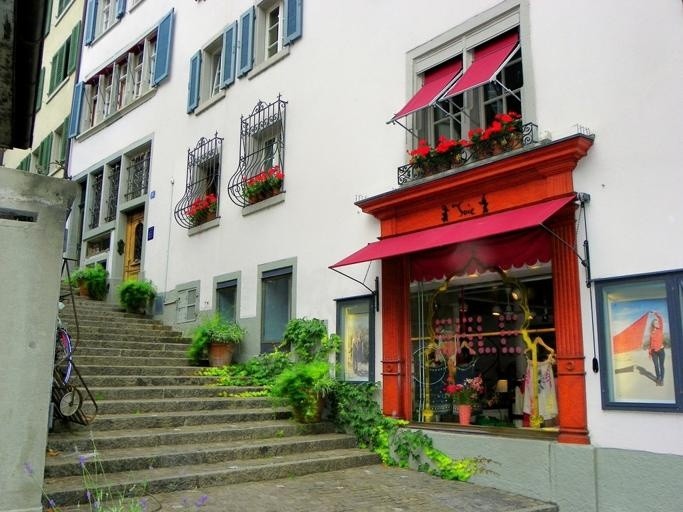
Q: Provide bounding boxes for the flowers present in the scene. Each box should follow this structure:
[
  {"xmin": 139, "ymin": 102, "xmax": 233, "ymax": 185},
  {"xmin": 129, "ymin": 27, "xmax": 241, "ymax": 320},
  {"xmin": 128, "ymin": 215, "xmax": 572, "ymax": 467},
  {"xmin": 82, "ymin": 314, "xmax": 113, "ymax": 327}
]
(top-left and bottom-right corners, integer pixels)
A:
[
  {"xmin": 406, "ymin": 111, "xmax": 522, "ymax": 167},
  {"xmin": 444, "ymin": 383, "xmax": 478, "ymax": 406},
  {"xmin": 181, "ymin": 192, "xmax": 219, "ymax": 225},
  {"xmin": 238, "ymin": 166, "xmax": 285, "ymax": 196}
]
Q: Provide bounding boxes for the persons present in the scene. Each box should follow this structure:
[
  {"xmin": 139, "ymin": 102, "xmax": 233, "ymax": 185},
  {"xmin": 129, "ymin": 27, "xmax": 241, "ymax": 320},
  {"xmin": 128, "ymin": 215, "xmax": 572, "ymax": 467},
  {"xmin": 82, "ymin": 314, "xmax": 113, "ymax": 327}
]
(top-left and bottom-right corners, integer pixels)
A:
[{"xmin": 648, "ymin": 311, "xmax": 667, "ymax": 387}]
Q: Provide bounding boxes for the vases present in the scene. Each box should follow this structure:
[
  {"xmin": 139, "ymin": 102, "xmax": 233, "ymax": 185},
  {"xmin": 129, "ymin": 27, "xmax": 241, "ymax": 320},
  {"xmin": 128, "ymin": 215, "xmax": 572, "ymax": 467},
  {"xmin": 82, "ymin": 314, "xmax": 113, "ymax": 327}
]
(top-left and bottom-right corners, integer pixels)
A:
[
  {"xmin": 457, "ymin": 403, "xmax": 474, "ymax": 427},
  {"xmin": 421, "ymin": 152, "xmax": 464, "ymax": 176},
  {"xmin": 246, "ymin": 187, "xmax": 279, "ymax": 206}
]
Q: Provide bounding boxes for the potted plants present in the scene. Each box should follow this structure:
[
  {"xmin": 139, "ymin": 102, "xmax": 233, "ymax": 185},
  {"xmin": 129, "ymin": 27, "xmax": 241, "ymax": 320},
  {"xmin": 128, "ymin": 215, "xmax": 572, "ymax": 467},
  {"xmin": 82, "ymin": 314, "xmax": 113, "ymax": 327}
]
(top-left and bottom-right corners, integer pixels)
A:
[
  {"xmin": 114, "ymin": 277, "xmax": 156, "ymax": 316},
  {"xmin": 61, "ymin": 262, "xmax": 108, "ymax": 301},
  {"xmin": 272, "ymin": 356, "xmax": 337, "ymax": 426},
  {"xmin": 185, "ymin": 311, "xmax": 248, "ymax": 371}
]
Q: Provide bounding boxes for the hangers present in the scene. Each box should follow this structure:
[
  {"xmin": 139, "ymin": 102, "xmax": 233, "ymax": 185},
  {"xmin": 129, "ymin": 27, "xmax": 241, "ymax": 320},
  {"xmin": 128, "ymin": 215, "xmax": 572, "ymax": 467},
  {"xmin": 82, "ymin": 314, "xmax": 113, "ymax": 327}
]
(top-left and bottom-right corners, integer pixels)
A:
[
  {"xmin": 521, "ymin": 329, "xmax": 555, "ymax": 355},
  {"xmin": 454, "ymin": 337, "xmax": 476, "ymax": 356}
]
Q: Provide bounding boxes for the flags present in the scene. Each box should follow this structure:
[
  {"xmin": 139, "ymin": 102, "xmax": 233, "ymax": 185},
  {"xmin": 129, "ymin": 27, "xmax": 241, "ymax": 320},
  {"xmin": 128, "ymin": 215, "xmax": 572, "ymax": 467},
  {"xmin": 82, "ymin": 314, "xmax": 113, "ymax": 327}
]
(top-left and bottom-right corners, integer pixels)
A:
[{"xmin": 612, "ymin": 313, "xmax": 648, "ymax": 354}]
